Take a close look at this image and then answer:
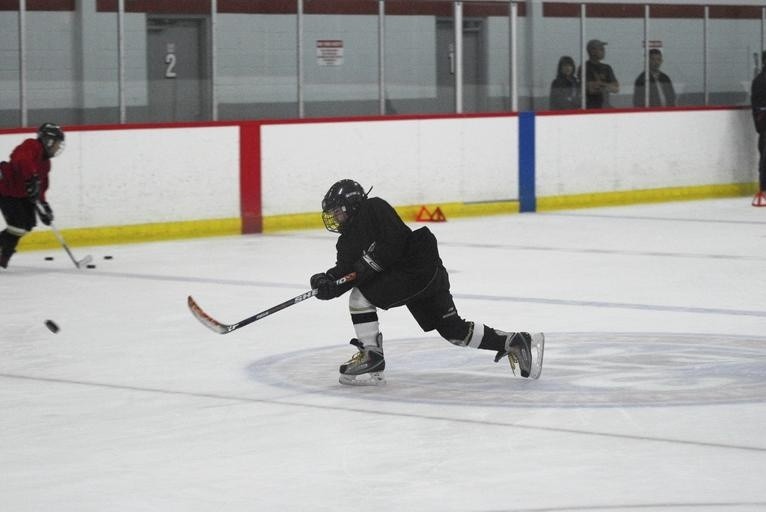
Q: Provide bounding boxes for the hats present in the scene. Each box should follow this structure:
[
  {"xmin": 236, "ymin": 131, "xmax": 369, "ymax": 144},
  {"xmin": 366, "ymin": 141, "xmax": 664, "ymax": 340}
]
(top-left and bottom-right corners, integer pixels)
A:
[{"xmin": 587, "ymin": 39, "xmax": 606, "ymax": 50}]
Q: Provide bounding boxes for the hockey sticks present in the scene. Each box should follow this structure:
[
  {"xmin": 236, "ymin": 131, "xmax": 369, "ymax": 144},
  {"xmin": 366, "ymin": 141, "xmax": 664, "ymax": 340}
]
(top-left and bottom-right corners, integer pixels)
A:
[
  {"xmin": 188, "ymin": 272, "xmax": 356, "ymax": 333},
  {"xmin": 34, "ymin": 199, "xmax": 93, "ymax": 268}
]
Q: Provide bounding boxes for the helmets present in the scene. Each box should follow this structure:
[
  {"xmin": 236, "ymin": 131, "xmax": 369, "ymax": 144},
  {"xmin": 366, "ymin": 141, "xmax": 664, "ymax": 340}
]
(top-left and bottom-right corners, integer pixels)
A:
[
  {"xmin": 38, "ymin": 123, "xmax": 65, "ymax": 157},
  {"xmin": 321, "ymin": 179, "xmax": 365, "ymax": 233}
]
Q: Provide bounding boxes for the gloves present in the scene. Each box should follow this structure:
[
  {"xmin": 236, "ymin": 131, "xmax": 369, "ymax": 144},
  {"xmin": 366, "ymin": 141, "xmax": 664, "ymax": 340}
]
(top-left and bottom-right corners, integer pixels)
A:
[
  {"xmin": 311, "ymin": 268, "xmax": 351, "ymax": 299},
  {"xmin": 37, "ymin": 202, "xmax": 53, "ymax": 226},
  {"xmin": 26, "ymin": 177, "xmax": 41, "ymax": 201}
]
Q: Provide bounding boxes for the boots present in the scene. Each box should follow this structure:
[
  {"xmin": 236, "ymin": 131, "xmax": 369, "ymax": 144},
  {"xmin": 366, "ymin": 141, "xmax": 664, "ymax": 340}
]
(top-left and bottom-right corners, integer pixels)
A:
[
  {"xmin": 340, "ymin": 332, "xmax": 385, "ymax": 375},
  {"xmin": 492, "ymin": 330, "xmax": 531, "ymax": 378}
]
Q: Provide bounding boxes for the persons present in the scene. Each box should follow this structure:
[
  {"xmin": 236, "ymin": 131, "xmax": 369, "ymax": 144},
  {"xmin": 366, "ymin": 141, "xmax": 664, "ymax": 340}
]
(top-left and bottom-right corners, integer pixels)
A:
[
  {"xmin": 751, "ymin": 51, "xmax": 766, "ymax": 206},
  {"xmin": 575, "ymin": 39, "xmax": 619, "ymax": 109},
  {"xmin": 549, "ymin": 56, "xmax": 581, "ymax": 111},
  {"xmin": 634, "ymin": 49, "xmax": 676, "ymax": 107},
  {"xmin": 0, "ymin": 123, "xmax": 64, "ymax": 268},
  {"xmin": 310, "ymin": 180, "xmax": 532, "ymax": 379}
]
